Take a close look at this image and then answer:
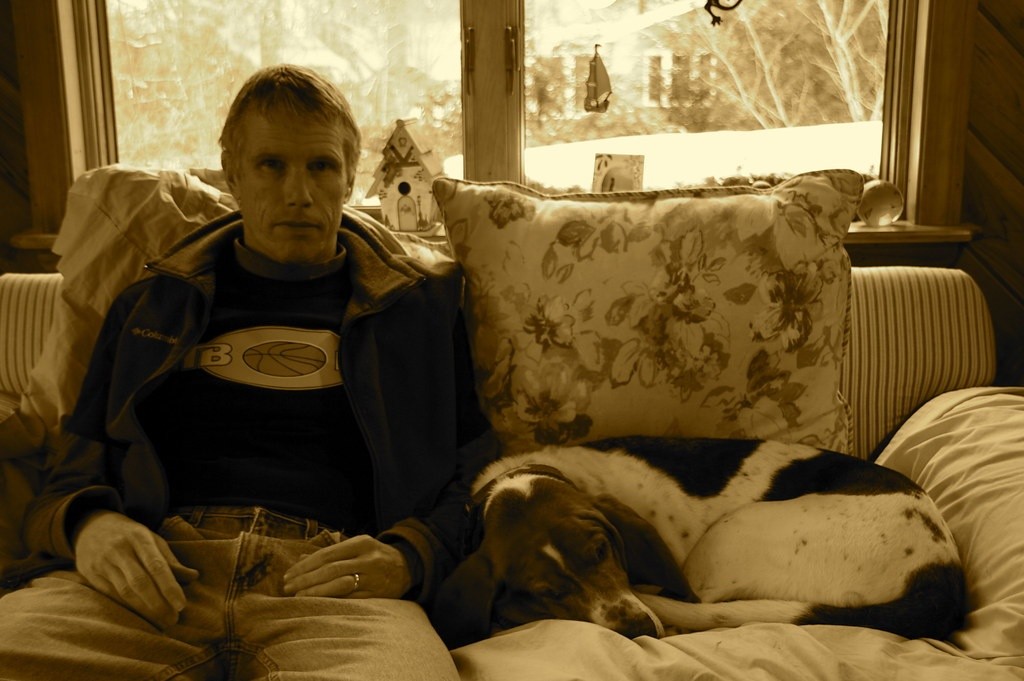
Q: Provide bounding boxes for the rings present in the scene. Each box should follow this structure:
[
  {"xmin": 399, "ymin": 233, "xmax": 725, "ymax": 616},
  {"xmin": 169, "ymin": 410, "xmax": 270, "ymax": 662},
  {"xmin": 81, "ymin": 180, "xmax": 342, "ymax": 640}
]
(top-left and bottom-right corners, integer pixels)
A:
[{"xmin": 354, "ymin": 574, "xmax": 360, "ymax": 590}]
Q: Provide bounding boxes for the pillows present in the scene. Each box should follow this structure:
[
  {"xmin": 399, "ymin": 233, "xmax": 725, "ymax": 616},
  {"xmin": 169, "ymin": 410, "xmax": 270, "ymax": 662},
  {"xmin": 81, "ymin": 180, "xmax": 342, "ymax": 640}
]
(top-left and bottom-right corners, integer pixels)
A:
[{"xmin": 388, "ymin": 170, "xmax": 866, "ymax": 459}]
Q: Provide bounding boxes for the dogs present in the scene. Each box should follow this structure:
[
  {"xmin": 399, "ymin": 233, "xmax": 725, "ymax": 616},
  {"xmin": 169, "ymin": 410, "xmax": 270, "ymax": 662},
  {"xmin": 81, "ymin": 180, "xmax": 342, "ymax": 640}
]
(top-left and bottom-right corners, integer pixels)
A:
[{"xmin": 429, "ymin": 432, "xmax": 965, "ymax": 650}]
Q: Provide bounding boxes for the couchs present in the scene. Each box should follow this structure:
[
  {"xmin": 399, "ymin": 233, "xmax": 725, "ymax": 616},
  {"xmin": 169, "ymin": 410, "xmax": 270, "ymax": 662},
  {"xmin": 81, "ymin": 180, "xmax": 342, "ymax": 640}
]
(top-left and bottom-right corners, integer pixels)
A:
[{"xmin": 0, "ymin": 267, "xmax": 1024, "ymax": 680}]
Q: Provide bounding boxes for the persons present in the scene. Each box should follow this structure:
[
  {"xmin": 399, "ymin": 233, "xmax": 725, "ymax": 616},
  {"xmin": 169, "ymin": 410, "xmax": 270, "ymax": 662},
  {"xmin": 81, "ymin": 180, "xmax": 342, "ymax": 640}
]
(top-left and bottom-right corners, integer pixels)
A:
[{"xmin": 1, "ymin": 64, "xmax": 504, "ymax": 681}]
